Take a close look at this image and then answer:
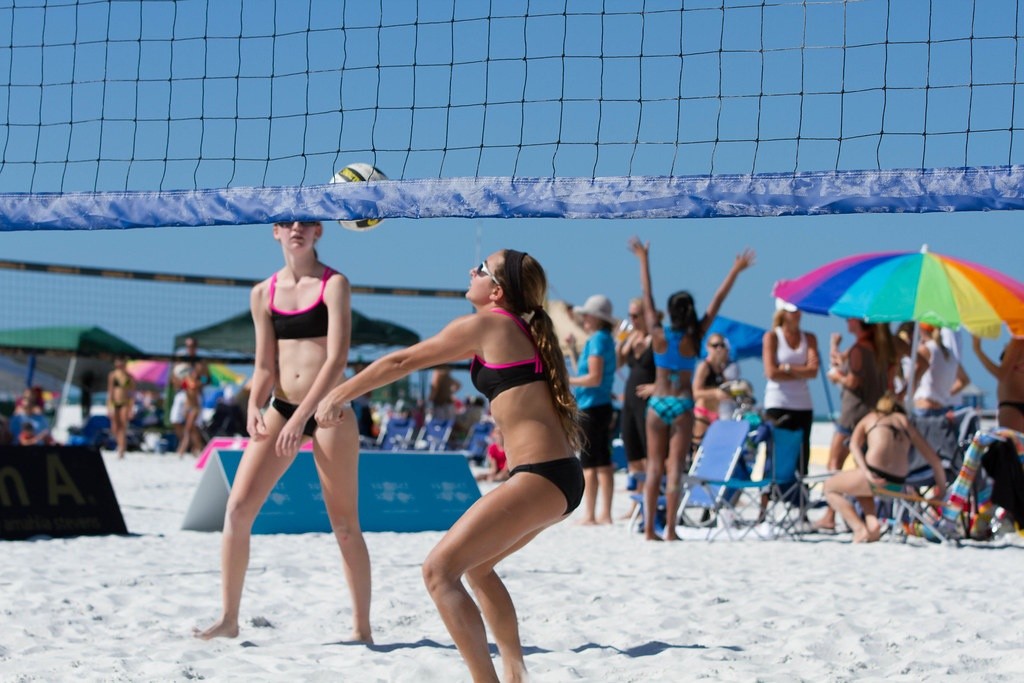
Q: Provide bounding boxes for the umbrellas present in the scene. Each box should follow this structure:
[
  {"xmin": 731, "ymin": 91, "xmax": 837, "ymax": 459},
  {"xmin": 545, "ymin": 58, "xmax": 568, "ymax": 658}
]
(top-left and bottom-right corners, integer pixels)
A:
[
  {"xmin": 773, "ymin": 243, "xmax": 1024, "ymax": 417},
  {"xmin": 127, "ymin": 359, "xmax": 246, "ymax": 390}
]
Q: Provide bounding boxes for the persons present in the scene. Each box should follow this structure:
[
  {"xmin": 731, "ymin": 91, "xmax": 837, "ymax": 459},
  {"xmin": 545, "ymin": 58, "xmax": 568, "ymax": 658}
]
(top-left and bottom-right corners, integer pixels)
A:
[
  {"xmin": 430, "ymin": 369, "xmax": 459, "ymax": 418},
  {"xmin": 816, "ymin": 318, "xmax": 929, "ymax": 528},
  {"xmin": 106, "ymin": 356, "xmax": 137, "ymax": 456},
  {"xmin": 692, "ymin": 334, "xmax": 732, "ymax": 453},
  {"xmin": 172, "ymin": 336, "xmax": 210, "ymax": 457},
  {"xmin": 564, "ymin": 294, "xmax": 618, "ymax": 525},
  {"xmin": 824, "ymin": 395, "xmax": 946, "ymax": 544},
  {"xmin": 896, "ymin": 322, "xmax": 969, "ymax": 417},
  {"xmin": 14, "ymin": 389, "xmax": 49, "ymax": 444},
  {"xmin": 477, "ymin": 426, "xmax": 511, "ymax": 482},
  {"xmin": 973, "ymin": 337, "xmax": 1024, "ymax": 433},
  {"xmin": 191, "ymin": 221, "xmax": 373, "ymax": 641},
  {"xmin": 616, "ymin": 298, "xmax": 656, "ymax": 522},
  {"xmin": 762, "ymin": 296, "xmax": 818, "ymax": 533},
  {"xmin": 352, "ymin": 361, "xmax": 374, "ymax": 436},
  {"xmin": 313, "ymin": 250, "xmax": 586, "ymax": 683},
  {"xmin": 625, "ymin": 236, "xmax": 756, "ymax": 541}
]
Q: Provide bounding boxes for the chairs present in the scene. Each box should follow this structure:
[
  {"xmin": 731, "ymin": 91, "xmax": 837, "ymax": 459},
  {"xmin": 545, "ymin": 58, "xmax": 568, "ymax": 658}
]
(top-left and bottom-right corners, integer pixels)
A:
[
  {"xmin": 356, "ymin": 417, "xmax": 493, "ymax": 467},
  {"xmin": 623, "ymin": 413, "xmax": 1023, "ymax": 546},
  {"xmin": 66, "ymin": 413, "xmax": 113, "ymax": 448}
]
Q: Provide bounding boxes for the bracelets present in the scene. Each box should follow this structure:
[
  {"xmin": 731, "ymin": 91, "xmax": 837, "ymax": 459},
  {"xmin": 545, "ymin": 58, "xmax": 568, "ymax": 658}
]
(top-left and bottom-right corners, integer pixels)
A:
[{"xmin": 784, "ymin": 363, "xmax": 790, "ymax": 374}]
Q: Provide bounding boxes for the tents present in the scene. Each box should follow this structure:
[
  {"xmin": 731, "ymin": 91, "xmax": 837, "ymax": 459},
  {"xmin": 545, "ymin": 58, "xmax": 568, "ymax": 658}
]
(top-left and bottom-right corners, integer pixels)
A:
[
  {"xmin": 0, "ymin": 325, "xmax": 144, "ymax": 404},
  {"xmin": 174, "ymin": 298, "xmax": 419, "ymax": 356}
]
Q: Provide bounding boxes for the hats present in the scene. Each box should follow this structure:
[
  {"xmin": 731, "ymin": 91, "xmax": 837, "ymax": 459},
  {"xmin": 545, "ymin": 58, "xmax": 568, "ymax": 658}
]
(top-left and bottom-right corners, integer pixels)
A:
[
  {"xmin": 573, "ymin": 295, "xmax": 618, "ymax": 325},
  {"xmin": 775, "ymin": 296, "xmax": 798, "ymax": 313}
]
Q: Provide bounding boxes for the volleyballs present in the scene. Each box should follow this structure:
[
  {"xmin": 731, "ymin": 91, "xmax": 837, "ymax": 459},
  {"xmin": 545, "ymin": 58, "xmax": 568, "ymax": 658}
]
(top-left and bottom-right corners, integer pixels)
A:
[{"xmin": 327, "ymin": 163, "xmax": 389, "ymax": 230}]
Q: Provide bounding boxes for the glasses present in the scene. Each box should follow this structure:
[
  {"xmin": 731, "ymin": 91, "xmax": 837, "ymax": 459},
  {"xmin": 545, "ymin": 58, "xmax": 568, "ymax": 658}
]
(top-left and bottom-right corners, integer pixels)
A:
[
  {"xmin": 628, "ymin": 312, "xmax": 645, "ymax": 319},
  {"xmin": 275, "ymin": 220, "xmax": 321, "ymax": 228},
  {"xmin": 709, "ymin": 343, "xmax": 727, "ymax": 351},
  {"xmin": 477, "ymin": 262, "xmax": 507, "ymax": 300}
]
